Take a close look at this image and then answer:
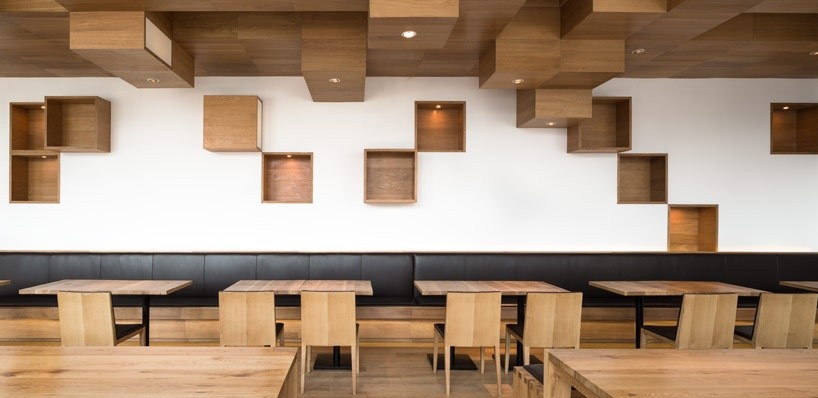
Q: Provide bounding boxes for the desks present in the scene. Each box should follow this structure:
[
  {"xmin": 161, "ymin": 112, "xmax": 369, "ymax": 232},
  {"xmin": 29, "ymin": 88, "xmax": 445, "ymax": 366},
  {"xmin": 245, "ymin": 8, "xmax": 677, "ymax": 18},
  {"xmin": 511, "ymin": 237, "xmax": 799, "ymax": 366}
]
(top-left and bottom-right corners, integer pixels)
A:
[
  {"xmin": 542, "ymin": 349, "xmax": 818, "ymax": 398},
  {"xmin": 19, "ymin": 279, "xmax": 194, "ymax": 346},
  {"xmin": 414, "ymin": 280, "xmax": 571, "ymax": 371},
  {"xmin": 222, "ymin": 279, "xmax": 374, "ymax": 370},
  {"xmin": 589, "ymin": 280, "xmax": 769, "ymax": 349},
  {"xmin": 0, "ymin": 345, "xmax": 300, "ymax": 398},
  {"xmin": 780, "ymin": 280, "xmax": 818, "ymax": 292}
]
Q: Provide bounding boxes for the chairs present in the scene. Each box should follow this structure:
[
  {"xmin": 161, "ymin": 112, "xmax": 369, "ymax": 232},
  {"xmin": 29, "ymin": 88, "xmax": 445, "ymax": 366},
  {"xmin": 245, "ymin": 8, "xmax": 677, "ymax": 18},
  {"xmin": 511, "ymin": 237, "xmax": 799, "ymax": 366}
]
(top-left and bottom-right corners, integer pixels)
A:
[
  {"xmin": 432, "ymin": 292, "xmax": 501, "ymax": 397},
  {"xmin": 300, "ymin": 290, "xmax": 360, "ymax": 396},
  {"xmin": 639, "ymin": 293, "xmax": 738, "ymax": 349},
  {"xmin": 57, "ymin": 291, "xmax": 146, "ymax": 346},
  {"xmin": 734, "ymin": 292, "xmax": 818, "ymax": 349},
  {"xmin": 505, "ymin": 291, "xmax": 583, "ymax": 375},
  {"xmin": 219, "ymin": 291, "xmax": 285, "ymax": 348}
]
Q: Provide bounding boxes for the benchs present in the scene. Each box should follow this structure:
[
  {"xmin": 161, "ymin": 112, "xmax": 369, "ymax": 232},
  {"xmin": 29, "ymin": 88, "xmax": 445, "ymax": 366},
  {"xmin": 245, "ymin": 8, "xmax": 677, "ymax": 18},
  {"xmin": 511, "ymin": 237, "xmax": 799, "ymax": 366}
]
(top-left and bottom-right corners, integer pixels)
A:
[
  {"xmin": 512, "ymin": 366, "xmax": 582, "ymax": 398},
  {"xmin": 0, "ymin": 251, "xmax": 818, "ymax": 344}
]
